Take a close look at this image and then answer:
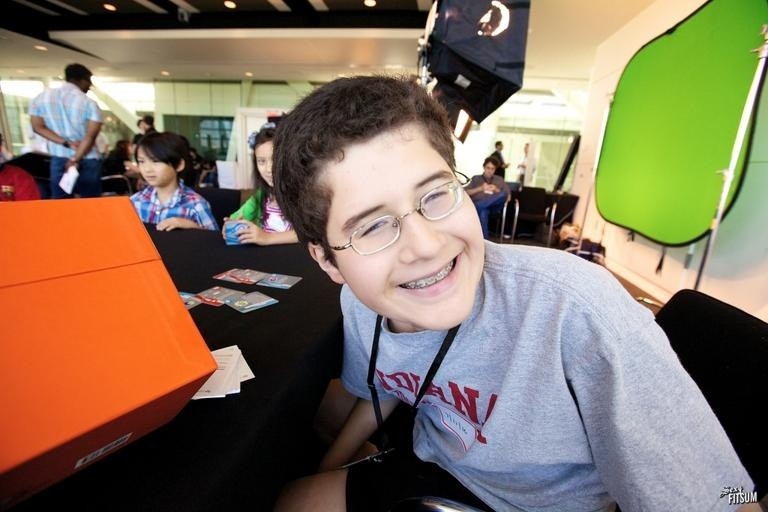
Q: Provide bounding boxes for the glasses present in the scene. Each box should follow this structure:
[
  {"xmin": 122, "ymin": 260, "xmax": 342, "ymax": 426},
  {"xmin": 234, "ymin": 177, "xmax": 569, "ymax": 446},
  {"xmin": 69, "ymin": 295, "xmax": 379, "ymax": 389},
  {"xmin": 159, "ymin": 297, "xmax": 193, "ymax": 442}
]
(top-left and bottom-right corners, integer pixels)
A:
[{"xmin": 317, "ymin": 169, "xmax": 473, "ymax": 256}]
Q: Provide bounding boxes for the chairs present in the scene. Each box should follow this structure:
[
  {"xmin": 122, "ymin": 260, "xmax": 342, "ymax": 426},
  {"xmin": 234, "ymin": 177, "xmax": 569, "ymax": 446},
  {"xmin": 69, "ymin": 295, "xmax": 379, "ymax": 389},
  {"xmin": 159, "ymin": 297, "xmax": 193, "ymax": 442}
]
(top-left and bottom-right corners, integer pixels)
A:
[{"xmin": 1, "ymin": 146, "xmax": 767, "ymax": 512}]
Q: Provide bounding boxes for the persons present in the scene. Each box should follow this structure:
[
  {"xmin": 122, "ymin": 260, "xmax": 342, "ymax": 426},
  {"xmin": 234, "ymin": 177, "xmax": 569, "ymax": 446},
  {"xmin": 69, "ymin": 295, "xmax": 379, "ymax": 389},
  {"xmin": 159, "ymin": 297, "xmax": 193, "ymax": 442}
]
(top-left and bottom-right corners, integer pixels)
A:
[
  {"xmin": 271, "ymin": 75, "xmax": 764, "ymax": 511},
  {"xmin": 28, "ymin": 60, "xmax": 218, "ymax": 238},
  {"xmin": 222, "ymin": 127, "xmax": 301, "ymax": 246},
  {"xmin": 313, "ymin": 376, "xmax": 380, "ymax": 468},
  {"xmin": 465, "ymin": 138, "xmax": 529, "ymax": 200}
]
